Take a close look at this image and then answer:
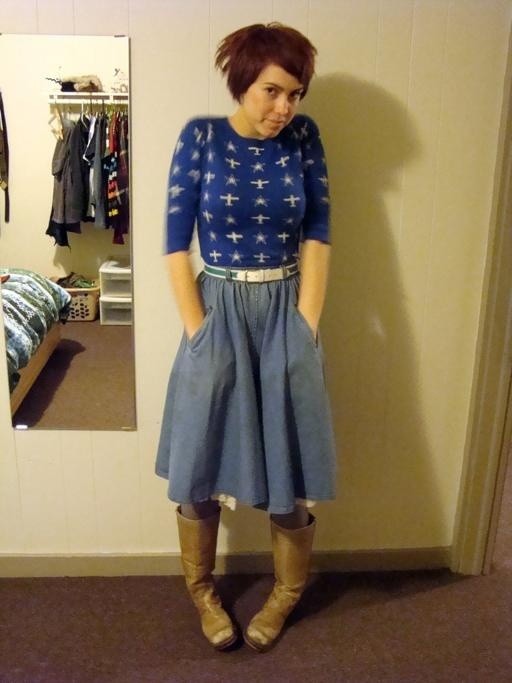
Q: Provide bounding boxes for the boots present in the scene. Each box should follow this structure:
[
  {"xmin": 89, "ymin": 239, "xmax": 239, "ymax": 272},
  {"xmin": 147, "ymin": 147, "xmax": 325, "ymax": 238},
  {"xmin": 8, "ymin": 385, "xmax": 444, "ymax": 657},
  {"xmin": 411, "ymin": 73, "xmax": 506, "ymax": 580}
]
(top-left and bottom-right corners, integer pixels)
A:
[
  {"xmin": 178, "ymin": 508, "xmax": 236, "ymax": 652},
  {"xmin": 244, "ymin": 514, "xmax": 315, "ymax": 652}
]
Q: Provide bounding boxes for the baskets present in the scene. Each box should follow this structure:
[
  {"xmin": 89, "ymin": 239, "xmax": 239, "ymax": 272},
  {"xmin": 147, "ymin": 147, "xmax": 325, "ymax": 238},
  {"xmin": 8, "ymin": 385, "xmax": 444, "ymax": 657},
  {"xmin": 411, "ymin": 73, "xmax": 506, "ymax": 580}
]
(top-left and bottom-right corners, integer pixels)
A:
[{"xmin": 48, "ymin": 277, "xmax": 99, "ymax": 320}]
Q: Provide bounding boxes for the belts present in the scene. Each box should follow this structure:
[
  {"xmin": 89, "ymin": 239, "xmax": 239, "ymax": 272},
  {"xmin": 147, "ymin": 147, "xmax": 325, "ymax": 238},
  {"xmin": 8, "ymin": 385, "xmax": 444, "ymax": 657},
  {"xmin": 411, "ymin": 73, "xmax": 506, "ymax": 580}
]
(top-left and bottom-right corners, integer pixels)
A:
[{"xmin": 204, "ymin": 263, "xmax": 298, "ymax": 284}]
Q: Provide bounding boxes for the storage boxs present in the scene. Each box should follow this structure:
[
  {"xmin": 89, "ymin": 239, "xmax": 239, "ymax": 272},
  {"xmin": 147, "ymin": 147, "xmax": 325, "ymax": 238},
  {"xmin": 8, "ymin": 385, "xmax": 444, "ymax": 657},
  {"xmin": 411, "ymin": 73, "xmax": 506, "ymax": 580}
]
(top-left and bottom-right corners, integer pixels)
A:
[{"xmin": 100, "ymin": 255, "xmax": 131, "ymax": 327}]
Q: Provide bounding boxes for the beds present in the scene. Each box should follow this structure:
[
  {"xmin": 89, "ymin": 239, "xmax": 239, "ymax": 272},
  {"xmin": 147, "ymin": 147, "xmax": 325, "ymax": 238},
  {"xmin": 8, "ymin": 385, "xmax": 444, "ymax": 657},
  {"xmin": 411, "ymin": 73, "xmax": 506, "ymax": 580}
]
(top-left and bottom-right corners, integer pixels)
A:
[{"xmin": 1, "ymin": 266, "xmax": 71, "ymax": 420}]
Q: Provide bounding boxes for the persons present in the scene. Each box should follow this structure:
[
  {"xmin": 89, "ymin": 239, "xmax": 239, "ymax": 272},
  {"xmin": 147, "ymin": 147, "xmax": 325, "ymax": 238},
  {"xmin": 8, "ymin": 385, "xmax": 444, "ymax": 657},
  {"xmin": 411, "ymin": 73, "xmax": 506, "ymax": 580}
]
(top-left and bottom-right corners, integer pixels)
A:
[{"xmin": 153, "ymin": 21, "xmax": 338, "ymax": 654}]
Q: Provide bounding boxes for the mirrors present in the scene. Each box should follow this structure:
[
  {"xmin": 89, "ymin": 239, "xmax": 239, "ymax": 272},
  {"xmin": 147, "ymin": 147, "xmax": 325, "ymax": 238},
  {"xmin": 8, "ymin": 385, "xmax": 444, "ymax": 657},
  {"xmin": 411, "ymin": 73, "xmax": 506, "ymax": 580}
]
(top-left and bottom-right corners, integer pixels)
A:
[{"xmin": 0, "ymin": 33, "xmax": 137, "ymax": 432}]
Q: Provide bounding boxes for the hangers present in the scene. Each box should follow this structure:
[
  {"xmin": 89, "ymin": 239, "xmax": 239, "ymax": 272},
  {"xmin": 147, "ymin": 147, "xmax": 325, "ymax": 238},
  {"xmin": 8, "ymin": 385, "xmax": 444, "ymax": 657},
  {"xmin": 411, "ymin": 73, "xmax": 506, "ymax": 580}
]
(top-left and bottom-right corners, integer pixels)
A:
[{"xmin": 49, "ymin": 94, "xmax": 124, "ymax": 138}]
